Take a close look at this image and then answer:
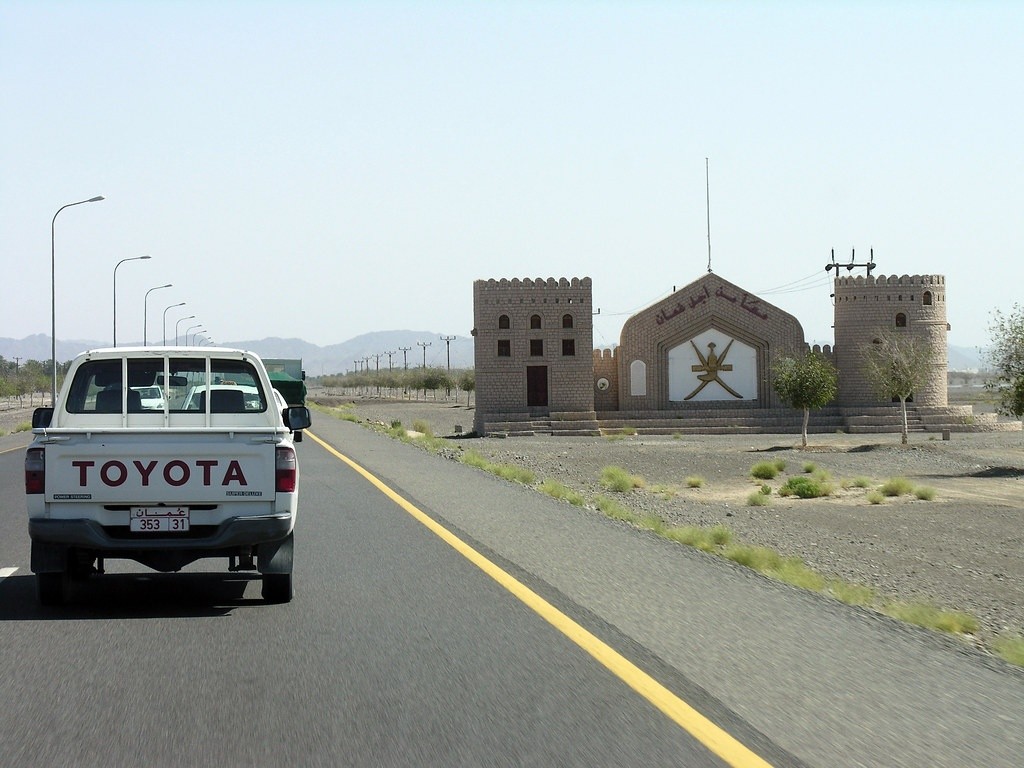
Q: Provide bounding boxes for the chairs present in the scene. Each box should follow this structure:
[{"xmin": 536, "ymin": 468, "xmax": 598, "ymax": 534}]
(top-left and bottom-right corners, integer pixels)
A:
[
  {"xmin": 199, "ymin": 390, "xmax": 245, "ymax": 413},
  {"xmin": 95, "ymin": 389, "xmax": 143, "ymax": 414}
]
